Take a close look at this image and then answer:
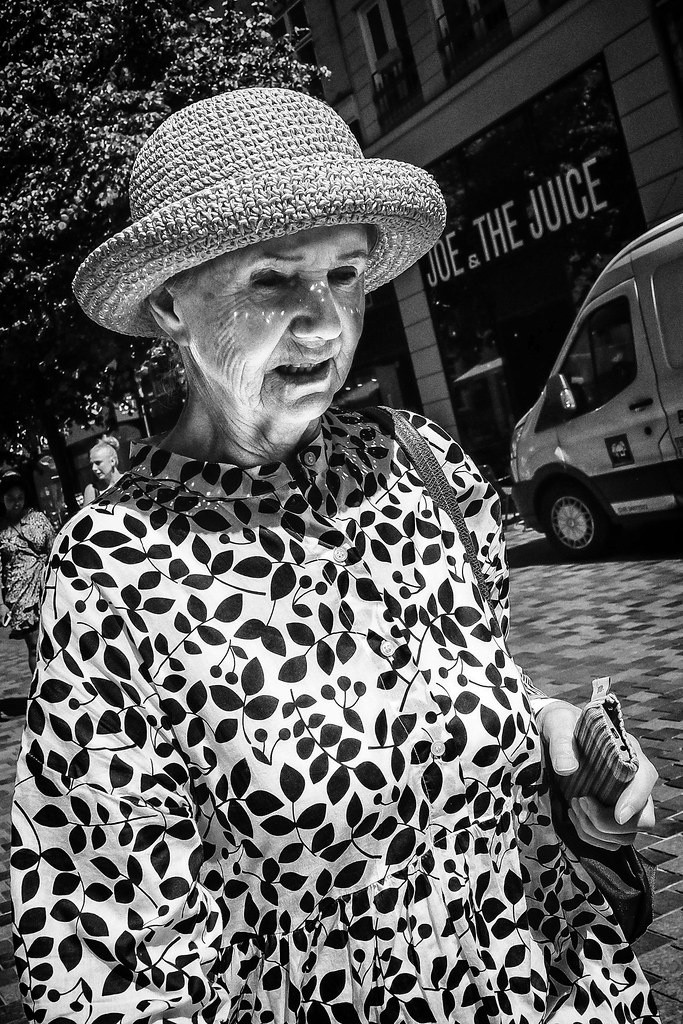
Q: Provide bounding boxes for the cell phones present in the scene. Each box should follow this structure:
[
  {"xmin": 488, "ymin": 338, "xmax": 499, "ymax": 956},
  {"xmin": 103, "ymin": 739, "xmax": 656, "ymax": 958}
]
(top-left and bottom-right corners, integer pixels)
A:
[{"xmin": 4, "ymin": 613, "xmax": 12, "ymax": 627}]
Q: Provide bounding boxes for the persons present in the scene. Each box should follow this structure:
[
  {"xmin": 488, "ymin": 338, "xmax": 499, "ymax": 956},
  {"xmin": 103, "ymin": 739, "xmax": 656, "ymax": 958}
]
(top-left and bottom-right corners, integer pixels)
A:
[{"xmin": 0, "ymin": 86, "xmax": 659, "ymax": 1024}]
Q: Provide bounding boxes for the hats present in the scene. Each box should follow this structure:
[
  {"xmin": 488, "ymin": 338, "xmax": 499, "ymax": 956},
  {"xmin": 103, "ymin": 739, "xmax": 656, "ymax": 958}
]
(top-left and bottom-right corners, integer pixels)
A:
[{"xmin": 71, "ymin": 88, "xmax": 447, "ymax": 337}]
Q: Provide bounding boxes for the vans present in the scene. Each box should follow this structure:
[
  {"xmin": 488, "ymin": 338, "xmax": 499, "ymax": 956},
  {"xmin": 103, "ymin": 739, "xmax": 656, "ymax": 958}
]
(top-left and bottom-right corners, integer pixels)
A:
[{"xmin": 501, "ymin": 208, "xmax": 683, "ymax": 565}]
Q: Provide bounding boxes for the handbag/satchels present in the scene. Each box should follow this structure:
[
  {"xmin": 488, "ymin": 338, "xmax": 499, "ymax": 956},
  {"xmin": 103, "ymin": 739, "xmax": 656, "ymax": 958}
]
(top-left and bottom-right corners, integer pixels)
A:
[{"xmin": 551, "ymin": 784, "xmax": 653, "ymax": 946}]
